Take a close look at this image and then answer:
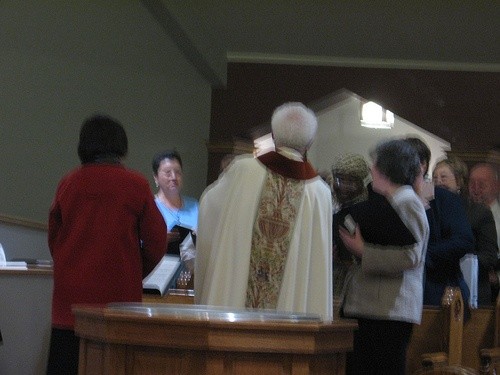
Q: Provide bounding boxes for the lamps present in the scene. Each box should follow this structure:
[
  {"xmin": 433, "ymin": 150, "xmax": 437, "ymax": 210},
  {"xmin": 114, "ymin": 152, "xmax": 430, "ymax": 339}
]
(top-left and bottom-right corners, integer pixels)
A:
[{"xmin": 362, "ymin": 101, "xmax": 394, "ymax": 131}]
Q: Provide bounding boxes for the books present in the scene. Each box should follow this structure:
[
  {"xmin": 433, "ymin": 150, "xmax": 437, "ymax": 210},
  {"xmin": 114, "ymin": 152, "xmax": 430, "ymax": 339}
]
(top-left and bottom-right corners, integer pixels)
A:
[
  {"xmin": 6, "ymin": 260, "xmax": 54, "ymax": 269},
  {"xmin": 333, "ymin": 195, "xmax": 417, "ymax": 259},
  {"xmin": 167, "ymin": 225, "xmax": 196, "ymax": 255},
  {"xmin": 141, "ymin": 254, "xmax": 183, "ymax": 295}
]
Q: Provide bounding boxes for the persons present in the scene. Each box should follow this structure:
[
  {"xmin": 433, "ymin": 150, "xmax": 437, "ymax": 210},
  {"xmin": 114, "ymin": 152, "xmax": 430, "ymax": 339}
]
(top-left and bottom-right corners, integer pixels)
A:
[
  {"xmin": 47, "ymin": 115, "xmax": 167, "ymax": 375},
  {"xmin": 153, "ymin": 150, "xmax": 198, "ymax": 245},
  {"xmin": 339, "ymin": 140, "xmax": 429, "ymax": 375},
  {"xmin": 404, "ymin": 136, "xmax": 500, "ymax": 308},
  {"xmin": 193, "ymin": 102, "xmax": 332, "ymax": 319}
]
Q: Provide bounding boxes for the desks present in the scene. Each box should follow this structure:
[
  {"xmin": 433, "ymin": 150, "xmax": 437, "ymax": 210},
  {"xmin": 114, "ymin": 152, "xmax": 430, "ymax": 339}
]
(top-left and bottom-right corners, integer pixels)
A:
[{"xmin": 71, "ymin": 302, "xmax": 360, "ymax": 375}]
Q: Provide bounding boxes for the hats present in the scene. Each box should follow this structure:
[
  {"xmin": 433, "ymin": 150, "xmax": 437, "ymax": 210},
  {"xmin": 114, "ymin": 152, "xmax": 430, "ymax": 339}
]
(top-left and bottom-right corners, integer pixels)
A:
[{"xmin": 332, "ymin": 152, "xmax": 369, "ymax": 179}]
